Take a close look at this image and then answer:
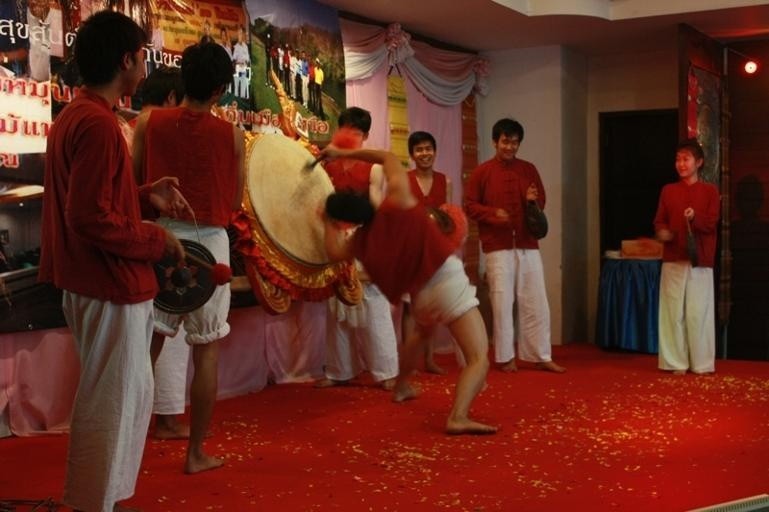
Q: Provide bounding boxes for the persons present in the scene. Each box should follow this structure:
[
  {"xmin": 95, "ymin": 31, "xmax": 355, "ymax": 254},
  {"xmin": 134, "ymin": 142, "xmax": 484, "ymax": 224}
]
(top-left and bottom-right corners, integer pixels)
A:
[
  {"xmin": 460, "ymin": 116, "xmax": 566, "ymax": 377},
  {"xmin": 652, "ymin": 138, "xmax": 719, "ymax": 376},
  {"xmin": 147, "ymin": 10, "xmax": 165, "ymax": 59},
  {"xmin": 131, "ymin": 38, "xmax": 248, "ymax": 474},
  {"xmin": 232, "ymin": 26, "xmax": 249, "ymax": 99},
  {"xmin": 403, "ymin": 128, "xmax": 454, "ymax": 374},
  {"xmin": 45, "ymin": 8, "xmax": 193, "ymax": 510},
  {"xmin": 311, "ymin": 106, "xmax": 399, "ymax": 391},
  {"xmin": 300, "ymin": 146, "xmax": 499, "ymax": 434},
  {"xmin": 218, "ymin": 26, "xmax": 232, "ymax": 61},
  {"xmin": 126, "ymin": 61, "xmax": 214, "ymax": 442},
  {"xmin": 199, "ymin": 21, "xmax": 217, "ymax": 42},
  {"xmin": 264, "ymin": 32, "xmax": 325, "ymax": 119},
  {"xmin": 0, "ymin": 0, "xmax": 29, "ymax": 81}
]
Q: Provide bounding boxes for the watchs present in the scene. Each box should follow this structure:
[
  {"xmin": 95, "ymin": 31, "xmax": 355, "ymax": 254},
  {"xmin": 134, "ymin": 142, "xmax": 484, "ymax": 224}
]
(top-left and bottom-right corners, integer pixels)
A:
[{"xmin": 1, "ymin": 53, "xmax": 9, "ymax": 65}]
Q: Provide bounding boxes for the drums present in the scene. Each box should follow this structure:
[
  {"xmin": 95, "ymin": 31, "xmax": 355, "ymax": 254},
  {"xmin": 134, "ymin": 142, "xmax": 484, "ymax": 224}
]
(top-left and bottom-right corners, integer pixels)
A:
[{"xmin": 229, "ymin": 133, "xmax": 363, "ymax": 314}]
